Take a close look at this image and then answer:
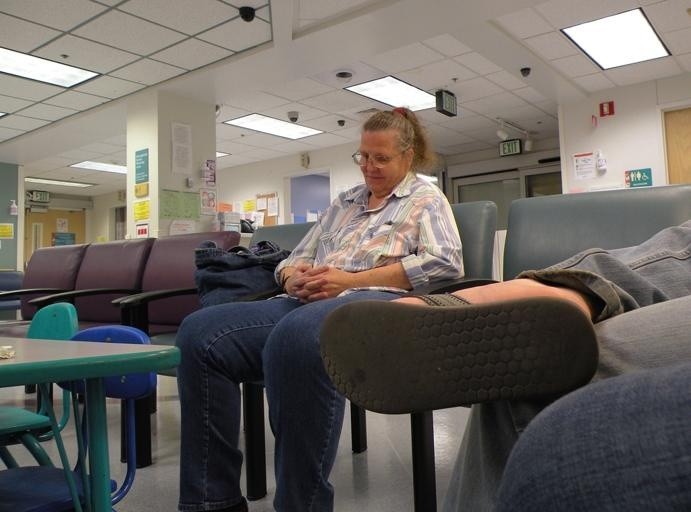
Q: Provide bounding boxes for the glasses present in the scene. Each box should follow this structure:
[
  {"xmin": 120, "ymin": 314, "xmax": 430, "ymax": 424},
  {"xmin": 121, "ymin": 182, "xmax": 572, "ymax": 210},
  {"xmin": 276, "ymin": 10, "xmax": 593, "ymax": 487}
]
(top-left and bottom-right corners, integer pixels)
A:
[{"xmin": 352, "ymin": 149, "xmax": 403, "ymax": 169}]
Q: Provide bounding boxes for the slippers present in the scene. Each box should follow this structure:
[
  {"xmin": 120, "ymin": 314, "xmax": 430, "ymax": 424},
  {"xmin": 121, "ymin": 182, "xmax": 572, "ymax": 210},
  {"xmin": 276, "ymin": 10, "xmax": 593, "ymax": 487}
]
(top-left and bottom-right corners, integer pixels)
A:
[{"xmin": 319, "ymin": 288, "xmax": 598, "ymax": 414}]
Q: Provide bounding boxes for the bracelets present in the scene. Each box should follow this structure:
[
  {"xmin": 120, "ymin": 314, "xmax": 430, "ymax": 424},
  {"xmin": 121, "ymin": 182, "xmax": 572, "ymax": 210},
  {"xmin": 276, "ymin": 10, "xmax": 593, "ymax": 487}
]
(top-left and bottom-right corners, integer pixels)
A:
[{"xmin": 280, "ymin": 274, "xmax": 289, "ymax": 292}]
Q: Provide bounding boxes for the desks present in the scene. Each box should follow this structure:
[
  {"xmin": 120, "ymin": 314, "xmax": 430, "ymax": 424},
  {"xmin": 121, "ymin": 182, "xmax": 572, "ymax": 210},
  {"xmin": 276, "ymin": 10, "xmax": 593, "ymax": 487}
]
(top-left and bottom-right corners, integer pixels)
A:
[{"xmin": 15, "ymin": 333, "xmax": 185, "ymax": 512}]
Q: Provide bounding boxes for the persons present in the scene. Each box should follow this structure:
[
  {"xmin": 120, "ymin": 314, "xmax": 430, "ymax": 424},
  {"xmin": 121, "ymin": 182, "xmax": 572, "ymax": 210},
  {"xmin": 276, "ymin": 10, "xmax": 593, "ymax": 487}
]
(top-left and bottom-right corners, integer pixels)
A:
[
  {"xmin": 323, "ymin": 216, "xmax": 690, "ymax": 509},
  {"xmin": 175, "ymin": 105, "xmax": 466, "ymax": 509}
]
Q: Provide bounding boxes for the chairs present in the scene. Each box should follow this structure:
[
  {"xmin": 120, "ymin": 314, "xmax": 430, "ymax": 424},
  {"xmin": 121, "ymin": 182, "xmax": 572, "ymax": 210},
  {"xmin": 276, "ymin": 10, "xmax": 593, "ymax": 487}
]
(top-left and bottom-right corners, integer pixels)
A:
[
  {"xmin": 243, "ymin": 197, "xmax": 499, "ymax": 510},
  {"xmin": 17, "ymin": 241, "xmax": 90, "ymax": 338},
  {"xmin": 15, "ymin": 302, "xmax": 78, "ymax": 466},
  {"xmin": 441, "ymin": 185, "xmax": 689, "ymax": 512},
  {"xmin": 129, "ymin": 219, "xmax": 319, "ymax": 500},
  {"xmin": 27, "ymin": 228, "xmax": 238, "ymax": 464},
  {"xmin": 15, "ymin": 232, "xmax": 153, "ymax": 340},
  {"xmin": 15, "ymin": 325, "xmax": 159, "ymax": 511}
]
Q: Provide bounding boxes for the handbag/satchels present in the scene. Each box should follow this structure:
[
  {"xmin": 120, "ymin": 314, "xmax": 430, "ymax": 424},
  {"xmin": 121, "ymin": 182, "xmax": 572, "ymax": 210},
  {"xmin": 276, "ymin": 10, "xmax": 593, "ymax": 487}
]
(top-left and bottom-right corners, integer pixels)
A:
[{"xmin": 193, "ymin": 239, "xmax": 291, "ymax": 306}]
